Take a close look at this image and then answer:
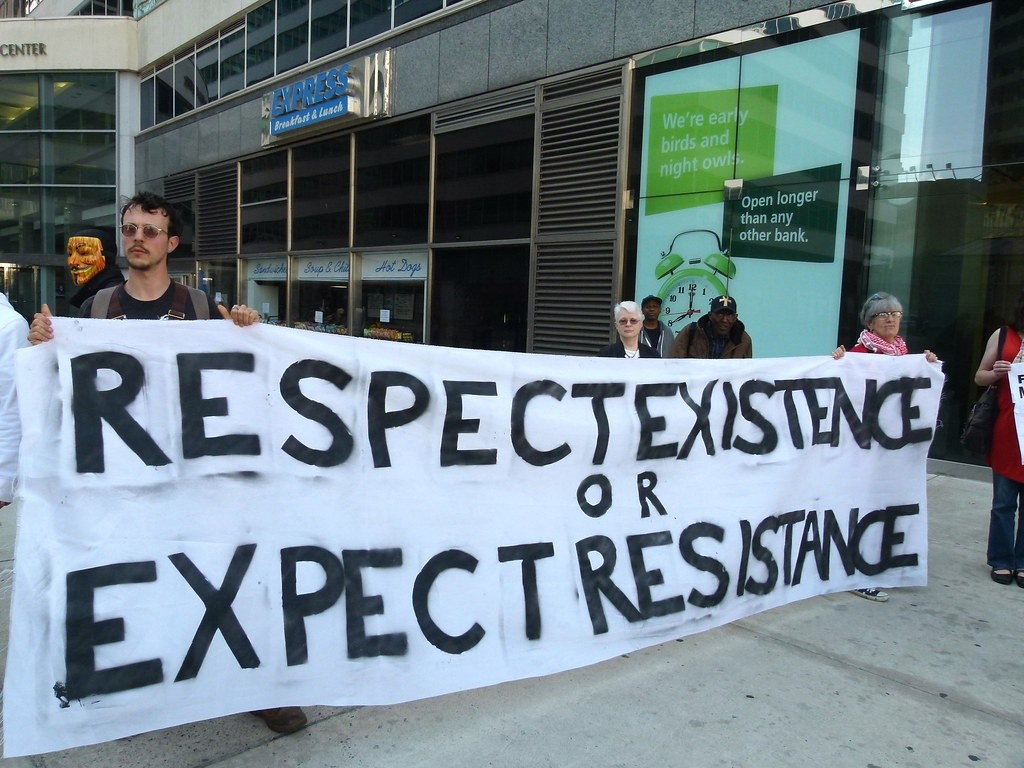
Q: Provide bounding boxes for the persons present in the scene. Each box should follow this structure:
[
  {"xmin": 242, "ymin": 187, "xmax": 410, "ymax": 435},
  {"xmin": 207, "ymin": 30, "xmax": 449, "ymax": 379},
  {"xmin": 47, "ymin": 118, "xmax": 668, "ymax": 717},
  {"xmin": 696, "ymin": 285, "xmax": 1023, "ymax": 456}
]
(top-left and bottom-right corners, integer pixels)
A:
[
  {"xmin": 832, "ymin": 292, "xmax": 938, "ymax": 601},
  {"xmin": 597, "ymin": 300, "xmax": 665, "ymax": 359},
  {"xmin": 668, "ymin": 294, "xmax": 752, "ymax": 359},
  {"xmin": 975, "ymin": 293, "xmax": 1024, "ymax": 588},
  {"xmin": 0, "ymin": 227, "xmax": 126, "ymax": 511},
  {"xmin": 638, "ymin": 295, "xmax": 674, "ymax": 359},
  {"xmin": 28, "ymin": 187, "xmax": 307, "ymax": 732}
]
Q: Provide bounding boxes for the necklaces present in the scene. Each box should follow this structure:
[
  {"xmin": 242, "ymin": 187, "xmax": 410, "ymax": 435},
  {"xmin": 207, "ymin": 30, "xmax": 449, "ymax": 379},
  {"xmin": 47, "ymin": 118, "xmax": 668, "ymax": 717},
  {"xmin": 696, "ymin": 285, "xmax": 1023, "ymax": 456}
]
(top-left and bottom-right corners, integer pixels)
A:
[{"xmin": 625, "ymin": 348, "xmax": 637, "ymax": 359}]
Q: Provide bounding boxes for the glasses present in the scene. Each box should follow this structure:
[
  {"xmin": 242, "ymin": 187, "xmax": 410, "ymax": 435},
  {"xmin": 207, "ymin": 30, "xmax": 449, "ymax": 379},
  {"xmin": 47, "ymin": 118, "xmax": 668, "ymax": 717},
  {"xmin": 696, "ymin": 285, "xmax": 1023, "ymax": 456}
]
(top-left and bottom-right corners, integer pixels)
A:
[
  {"xmin": 615, "ymin": 319, "xmax": 641, "ymax": 325},
  {"xmin": 119, "ymin": 224, "xmax": 169, "ymax": 239},
  {"xmin": 871, "ymin": 311, "xmax": 903, "ymax": 321}
]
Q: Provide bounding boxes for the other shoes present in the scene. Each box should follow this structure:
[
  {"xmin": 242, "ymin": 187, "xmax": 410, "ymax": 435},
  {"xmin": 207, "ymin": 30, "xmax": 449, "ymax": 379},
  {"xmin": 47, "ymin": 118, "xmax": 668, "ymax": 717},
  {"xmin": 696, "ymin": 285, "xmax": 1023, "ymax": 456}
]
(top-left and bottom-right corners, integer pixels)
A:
[
  {"xmin": 991, "ymin": 565, "xmax": 1015, "ymax": 585},
  {"xmin": 252, "ymin": 706, "xmax": 307, "ymax": 734},
  {"xmin": 1016, "ymin": 566, "xmax": 1024, "ymax": 589}
]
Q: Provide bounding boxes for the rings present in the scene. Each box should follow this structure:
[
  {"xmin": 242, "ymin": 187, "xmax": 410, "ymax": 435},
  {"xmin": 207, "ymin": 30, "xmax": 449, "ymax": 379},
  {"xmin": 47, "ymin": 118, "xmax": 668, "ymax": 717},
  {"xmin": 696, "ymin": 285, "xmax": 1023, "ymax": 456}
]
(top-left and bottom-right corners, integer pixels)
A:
[{"xmin": 232, "ymin": 305, "xmax": 240, "ymax": 311}]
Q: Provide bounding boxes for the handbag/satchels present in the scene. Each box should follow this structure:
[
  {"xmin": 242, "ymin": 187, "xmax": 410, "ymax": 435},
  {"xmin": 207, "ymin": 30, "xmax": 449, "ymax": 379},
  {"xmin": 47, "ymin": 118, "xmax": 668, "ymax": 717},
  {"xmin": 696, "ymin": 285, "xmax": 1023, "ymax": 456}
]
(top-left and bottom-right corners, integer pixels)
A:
[{"xmin": 960, "ymin": 325, "xmax": 1007, "ymax": 447}]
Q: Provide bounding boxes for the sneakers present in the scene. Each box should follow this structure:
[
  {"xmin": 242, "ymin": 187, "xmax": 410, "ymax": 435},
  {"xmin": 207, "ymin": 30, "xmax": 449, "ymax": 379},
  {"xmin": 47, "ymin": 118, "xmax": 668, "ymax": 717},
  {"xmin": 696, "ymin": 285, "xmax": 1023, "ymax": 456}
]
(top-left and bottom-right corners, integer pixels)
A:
[{"xmin": 849, "ymin": 588, "xmax": 890, "ymax": 602}]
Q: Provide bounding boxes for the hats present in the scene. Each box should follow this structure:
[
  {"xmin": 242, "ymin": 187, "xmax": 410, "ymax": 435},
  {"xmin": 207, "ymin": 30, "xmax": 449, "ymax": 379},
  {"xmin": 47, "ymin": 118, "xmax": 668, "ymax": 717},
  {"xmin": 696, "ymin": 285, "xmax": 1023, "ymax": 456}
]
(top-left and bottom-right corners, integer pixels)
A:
[
  {"xmin": 711, "ymin": 295, "xmax": 736, "ymax": 314},
  {"xmin": 642, "ymin": 295, "xmax": 662, "ymax": 310}
]
